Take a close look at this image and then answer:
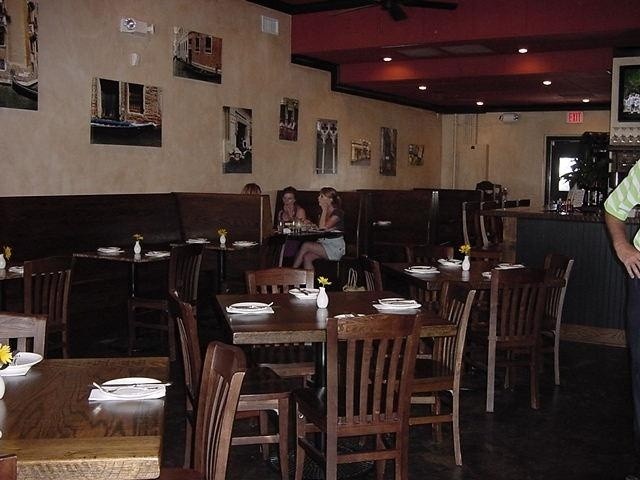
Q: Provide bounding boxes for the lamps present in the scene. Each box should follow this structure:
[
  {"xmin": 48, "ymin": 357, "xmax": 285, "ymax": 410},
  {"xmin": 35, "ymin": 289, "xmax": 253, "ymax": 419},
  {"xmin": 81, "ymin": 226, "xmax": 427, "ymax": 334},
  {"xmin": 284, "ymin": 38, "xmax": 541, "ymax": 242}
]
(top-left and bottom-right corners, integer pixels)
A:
[
  {"xmin": 121, "ymin": 18, "xmax": 156, "ymax": 38},
  {"xmin": 498, "ymin": 113, "xmax": 520, "ymax": 123}
]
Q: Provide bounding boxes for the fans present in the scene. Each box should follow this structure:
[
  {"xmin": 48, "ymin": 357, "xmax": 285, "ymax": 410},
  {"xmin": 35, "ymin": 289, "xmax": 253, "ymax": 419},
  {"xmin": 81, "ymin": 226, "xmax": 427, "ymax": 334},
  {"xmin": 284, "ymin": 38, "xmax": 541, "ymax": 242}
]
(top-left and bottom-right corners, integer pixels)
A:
[{"xmin": 344, "ymin": 0, "xmax": 459, "ymax": 21}]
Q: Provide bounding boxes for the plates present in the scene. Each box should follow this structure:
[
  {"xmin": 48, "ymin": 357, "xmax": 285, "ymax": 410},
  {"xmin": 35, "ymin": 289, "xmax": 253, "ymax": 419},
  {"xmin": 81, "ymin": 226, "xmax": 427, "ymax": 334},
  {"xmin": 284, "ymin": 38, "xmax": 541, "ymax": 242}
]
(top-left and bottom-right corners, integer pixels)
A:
[
  {"xmin": 481, "ymin": 261, "xmax": 524, "ymax": 278},
  {"xmin": 93, "ymin": 236, "xmax": 258, "ymax": 261},
  {"xmin": 436, "ymin": 257, "xmax": 462, "ymax": 266},
  {"xmin": 371, "ymin": 296, "xmax": 422, "ymax": 318},
  {"xmin": 288, "ymin": 288, "xmax": 323, "ymax": 298},
  {"xmin": 100, "ymin": 378, "xmax": 168, "ymax": 399},
  {"xmin": 0, "ymin": 351, "xmax": 42, "ymax": 370},
  {"xmin": 229, "ymin": 302, "xmax": 271, "ymax": 314},
  {"xmin": 410, "ymin": 264, "xmax": 438, "ymax": 273}
]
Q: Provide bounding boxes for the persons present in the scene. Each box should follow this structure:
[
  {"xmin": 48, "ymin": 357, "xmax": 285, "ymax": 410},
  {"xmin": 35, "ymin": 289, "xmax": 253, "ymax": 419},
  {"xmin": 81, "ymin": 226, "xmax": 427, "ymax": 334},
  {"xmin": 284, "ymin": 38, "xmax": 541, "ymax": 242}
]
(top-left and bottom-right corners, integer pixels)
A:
[
  {"xmin": 290, "ymin": 187, "xmax": 347, "ymax": 277},
  {"xmin": 602, "ymin": 157, "xmax": 640, "ymax": 478},
  {"xmin": 277, "ymin": 186, "xmax": 307, "ymax": 231},
  {"xmin": 242, "ymin": 183, "xmax": 262, "ymax": 195}
]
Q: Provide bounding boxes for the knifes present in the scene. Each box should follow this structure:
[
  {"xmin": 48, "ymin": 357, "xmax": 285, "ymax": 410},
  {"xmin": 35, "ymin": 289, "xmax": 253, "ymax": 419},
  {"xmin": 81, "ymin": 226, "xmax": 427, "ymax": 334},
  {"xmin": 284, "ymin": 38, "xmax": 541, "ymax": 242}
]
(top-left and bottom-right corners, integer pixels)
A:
[
  {"xmin": 1, "ymin": 348, "xmax": 20, "ymax": 369},
  {"xmin": 97, "ymin": 381, "xmax": 172, "ymax": 387}
]
